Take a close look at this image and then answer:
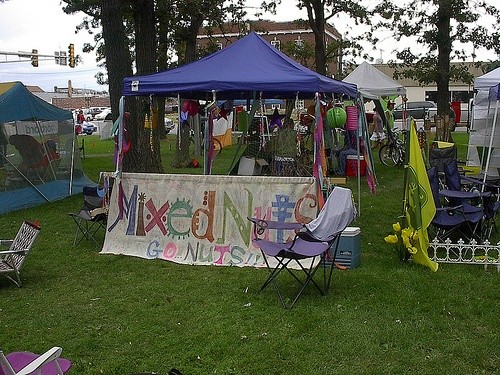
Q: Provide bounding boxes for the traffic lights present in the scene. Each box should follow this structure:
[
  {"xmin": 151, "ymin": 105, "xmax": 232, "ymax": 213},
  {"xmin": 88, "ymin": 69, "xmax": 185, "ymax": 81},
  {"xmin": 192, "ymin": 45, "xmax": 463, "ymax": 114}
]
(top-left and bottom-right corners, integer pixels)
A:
[{"xmin": 69, "ymin": 44, "xmax": 75, "ymax": 67}]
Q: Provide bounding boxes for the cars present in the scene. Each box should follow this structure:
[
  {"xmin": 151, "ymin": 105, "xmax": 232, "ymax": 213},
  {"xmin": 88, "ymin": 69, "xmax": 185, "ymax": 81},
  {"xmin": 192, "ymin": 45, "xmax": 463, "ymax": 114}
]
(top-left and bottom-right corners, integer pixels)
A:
[
  {"xmin": 63, "ymin": 107, "xmax": 113, "ymax": 135},
  {"xmin": 165, "ymin": 102, "xmax": 179, "ymax": 113},
  {"xmin": 425, "ymin": 108, "xmax": 438, "ymax": 127},
  {"xmin": 392, "ymin": 101, "xmax": 437, "ymax": 121},
  {"xmin": 164, "ymin": 116, "xmax": 175, "ymax": 134}
]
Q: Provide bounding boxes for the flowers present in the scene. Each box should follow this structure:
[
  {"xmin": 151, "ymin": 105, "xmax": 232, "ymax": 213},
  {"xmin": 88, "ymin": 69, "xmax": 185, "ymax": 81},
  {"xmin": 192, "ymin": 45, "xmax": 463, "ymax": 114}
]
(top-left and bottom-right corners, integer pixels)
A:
[
  {"xmin": 473, "ymin": 255, "xmax": 497, "ymax": 263},
  {"xmin": 382, "ymin": 222, "xmax": 422, "ymax": 263}
]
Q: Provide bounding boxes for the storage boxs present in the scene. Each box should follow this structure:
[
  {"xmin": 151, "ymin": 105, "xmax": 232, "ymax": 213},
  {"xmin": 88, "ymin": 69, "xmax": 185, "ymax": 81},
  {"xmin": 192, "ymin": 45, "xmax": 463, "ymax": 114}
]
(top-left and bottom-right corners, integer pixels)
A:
[
  {"xmin": 272, "ymin": 156, "xmax": 296, "ymax": 176},
  {"xmin": 346, "ymin": 154, "xmax": 366, "ymax": 178},
  {"xmin": 236, "ymin": 155, "xmax": 269, "ymax": 175},
  {"xmin": 323, "ymin": 227, "xmax": 362, "ymax": 271}
]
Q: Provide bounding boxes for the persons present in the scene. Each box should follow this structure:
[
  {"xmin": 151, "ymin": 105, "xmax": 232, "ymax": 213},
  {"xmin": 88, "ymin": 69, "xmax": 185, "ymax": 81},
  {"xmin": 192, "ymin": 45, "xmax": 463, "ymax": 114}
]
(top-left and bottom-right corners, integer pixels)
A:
[
  {"xmin": 33, "ymin": 140, "xmax": 60, "ymax": 182},
  {"xmin": 368, "ymin": 106, "xmax": 396, "ymax": 152},
  {"xmin": 8, "ymin": 134, "xmax": 46, "ymax": 181},
  {"xmin": 0, "ymin": 123, "xmax": 9, "ymax": 167},
  {"xmin": 75, "ymin": 110, "xmax": 85, "ymax": 134},
  {"xmin": 333, "ymin": 131, "xmax": 362, "ymax": 178},
  {"xmin": 263, "ymin": 118, "xmax": 311, "ymax": 176}
]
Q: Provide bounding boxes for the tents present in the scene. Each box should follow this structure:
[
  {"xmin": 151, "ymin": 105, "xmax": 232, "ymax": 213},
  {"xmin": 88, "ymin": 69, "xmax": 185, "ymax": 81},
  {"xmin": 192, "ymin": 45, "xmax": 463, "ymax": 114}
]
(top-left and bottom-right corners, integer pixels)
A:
[
  {"xmin": 119, "ymin": 30, "xmax": 360, "ymax": 218},
  {"xmin": 0, "ymin": 80, "xmax": 100, "ymax": 212},
  {"xmin": 341, "ymin": 61, "xmax": 407, "ymax": 100},
  {"xmin": 465, "ymin": 67, "xmax": 500, "ymax": 177}
]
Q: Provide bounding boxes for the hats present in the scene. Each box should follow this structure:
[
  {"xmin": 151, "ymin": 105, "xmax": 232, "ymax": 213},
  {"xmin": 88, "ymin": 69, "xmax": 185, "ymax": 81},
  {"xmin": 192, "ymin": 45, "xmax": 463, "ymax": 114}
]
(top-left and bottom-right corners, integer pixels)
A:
[
  {"xmin": 97, "ymin": 171, "xmax": 110, "ymax": 196},
  {"xmin": 387, "ymin": 100, "xmax": 396, "ymax": 104}
]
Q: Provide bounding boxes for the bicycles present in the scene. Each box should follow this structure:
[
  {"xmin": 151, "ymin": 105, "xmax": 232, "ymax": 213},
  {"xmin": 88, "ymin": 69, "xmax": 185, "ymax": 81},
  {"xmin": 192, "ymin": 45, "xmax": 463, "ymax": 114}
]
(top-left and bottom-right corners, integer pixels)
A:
[
  {"xmin": 185, "ymin": 134, "xmax": 222, "ymax": 156},
  {"xmin": 379, "ymin": 126, "xmax": 406, "ymax": 167}
]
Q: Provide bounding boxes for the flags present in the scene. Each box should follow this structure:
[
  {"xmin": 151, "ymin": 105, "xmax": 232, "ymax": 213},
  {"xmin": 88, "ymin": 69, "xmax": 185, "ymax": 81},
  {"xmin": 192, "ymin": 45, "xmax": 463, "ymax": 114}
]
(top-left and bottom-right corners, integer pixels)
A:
[{"xmin": 402, "ymin": 117, "xmax": 439, "ymax": 273}]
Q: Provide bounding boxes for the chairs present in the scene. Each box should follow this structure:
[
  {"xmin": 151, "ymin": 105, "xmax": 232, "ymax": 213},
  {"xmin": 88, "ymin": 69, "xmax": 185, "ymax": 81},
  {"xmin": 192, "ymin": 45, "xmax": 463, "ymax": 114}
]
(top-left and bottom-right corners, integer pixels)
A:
[
  {"xmin": 425, "ymin": 141, "xmax": 500, "ymax": 259},
  {"xmin": 4, "ymin": 154, "xmax": 60, "ymax": 190},
  {"xmin": 245, "ymin": 185, "xmax": 359, "ymax": 310},
  {"xmin": 0, "ymin": 220, "xmax": 41, "ymax": 287},
  {"xmin": 65, "ymin": 186, "xmax": 108, "ymax": 249},
  {"xmin": 0, "ymin": 345, "xmax": 72, "ymax": 375}
]
